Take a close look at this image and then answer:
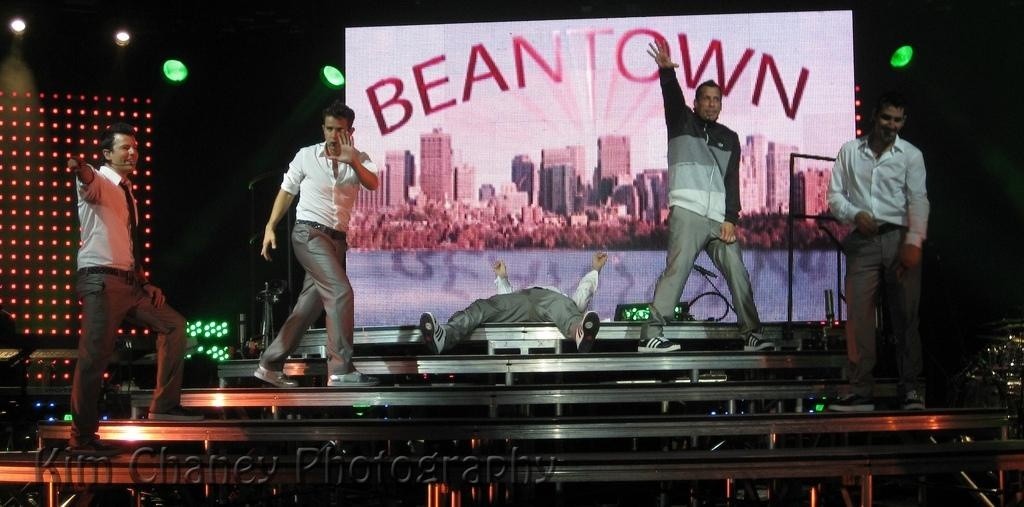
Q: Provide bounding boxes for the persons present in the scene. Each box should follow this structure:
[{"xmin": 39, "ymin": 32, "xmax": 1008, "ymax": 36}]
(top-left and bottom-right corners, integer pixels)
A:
[
  {"xmin": 254, "ymin": 105, "xmax": 380, "ymax": 387},
  {"xmin": 827, "ymin": 91, "xmax": 929, "ymax": 413},
  {"xmin": 636, "ymin": 39, "xmax": 775, "ymax": 353},
  {"xmin": 67, "ymin": 127, "xmax": 205, "ymax": 456},
  {"xmin": 420, "ymin": 253, "xmax": 607, "ymax": 355}
]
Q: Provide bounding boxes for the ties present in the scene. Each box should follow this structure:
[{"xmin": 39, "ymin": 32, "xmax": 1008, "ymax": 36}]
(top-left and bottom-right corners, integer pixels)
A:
[{"xmin": 119, "ymin": 182, "xmax": 142, "ymax": 270}]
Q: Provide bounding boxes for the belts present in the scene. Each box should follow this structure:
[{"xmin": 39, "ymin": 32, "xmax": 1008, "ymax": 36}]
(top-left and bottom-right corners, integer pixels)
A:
[
  {"xmin": 77, "ymin": 266, "xmax": 136, "ymax": 280},
  {"xmin": 859, "ymin": 222, "xmax": 901, "ymax": 234},
  {"xmin": 296, "ymin": 220, "xmax": 346, "ymax": 240}
]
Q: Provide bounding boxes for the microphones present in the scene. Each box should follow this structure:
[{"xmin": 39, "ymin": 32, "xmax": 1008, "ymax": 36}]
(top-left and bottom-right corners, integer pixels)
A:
[{"xmin": 693, "ymin": 264, "xmax": 719, "ymax": 280}]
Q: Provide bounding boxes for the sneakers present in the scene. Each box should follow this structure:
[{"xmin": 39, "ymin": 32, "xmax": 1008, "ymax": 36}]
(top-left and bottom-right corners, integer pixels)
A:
[
  {"xmin": 638, "ymin": 335, "xmax": 681, "ymax": 352},
  {"xmin": 901, "ymin": 393, "xmax": 925, "ymax": 409},
  {"xmin": 327, "ymin": 370, "xmax": 379, "ymax": 387},
  {"xmin": 576, "ymin": 311, "xmax": 600, "ymax": 353},
  {"xmin": 148, "ymin": 406, "xmax": 204, "ymax": 421},
  {"xmin": 830, "ymin": 395, "xmax": 875, "ymax": 412},
  {"xmin": 71, "ymin": 441, "xmax": 122, "ymax": 456},
  {"xmin": 744, "ymin": 333, "xmax": 774, "ymax": 351},
  {"xmin": 254, "ymin": 365, "xmax": 298, "ymax": 387},
  {"xmin": 420, "ymin": 313, "xmax": 446, "ymax": 355}
]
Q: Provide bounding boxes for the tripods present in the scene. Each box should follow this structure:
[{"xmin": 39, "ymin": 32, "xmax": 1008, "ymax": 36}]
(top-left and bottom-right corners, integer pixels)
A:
[{"xmin": 259, "ymin": 302, "xmax": 275, "ymax": 359}]
